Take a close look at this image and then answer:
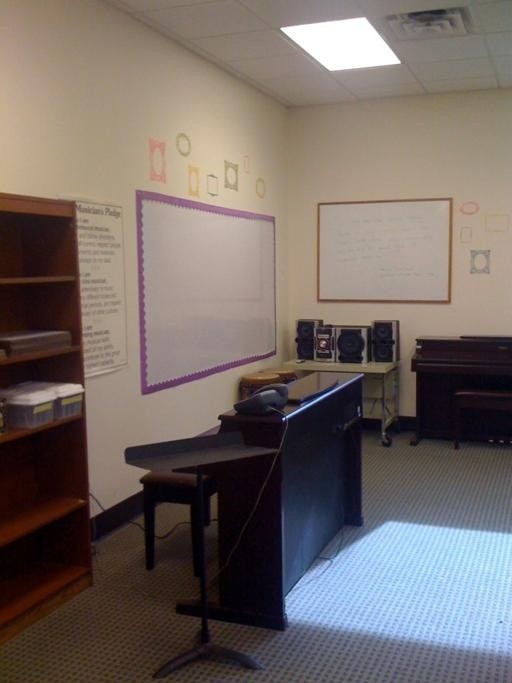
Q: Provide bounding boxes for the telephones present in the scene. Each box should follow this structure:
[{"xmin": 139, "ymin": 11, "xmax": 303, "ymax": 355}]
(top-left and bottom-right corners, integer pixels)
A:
[{"xmin": 233, "ymin": 383, "xmax": 289, "ymax": 415}]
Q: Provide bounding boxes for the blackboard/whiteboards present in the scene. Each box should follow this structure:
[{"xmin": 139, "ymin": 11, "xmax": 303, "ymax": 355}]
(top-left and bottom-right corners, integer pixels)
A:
[{"xmin": 317, "ymin": 197, "xmax": 453, "ymax": 303}]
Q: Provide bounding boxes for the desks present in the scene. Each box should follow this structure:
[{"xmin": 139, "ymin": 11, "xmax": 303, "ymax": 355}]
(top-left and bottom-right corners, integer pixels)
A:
[
  {"xmin": 176, "ymin": 371, "xmax": 365, "ymax": 630},
  {"xmin": 283, "ymin": 358, "xmax": 401, "ymax": 446}
]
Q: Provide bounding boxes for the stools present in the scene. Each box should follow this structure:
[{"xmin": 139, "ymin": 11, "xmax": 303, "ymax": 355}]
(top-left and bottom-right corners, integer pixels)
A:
[
  {"xmin": 239, "ymin": 373, "xmax": 280, "ymax": 400},
  {"xmin": 259, "ymin": 368, "xmax": 298, "ymax": 380}
]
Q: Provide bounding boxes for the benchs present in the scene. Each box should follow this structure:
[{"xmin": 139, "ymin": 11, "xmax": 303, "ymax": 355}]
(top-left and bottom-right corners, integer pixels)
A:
[
  {"xmin": 139, "ymin": 424, "xmax": 221, "ymax": 578},
  {"xmin": 451, "ymin": 389, "xmax": 512, "ymax": 449}
]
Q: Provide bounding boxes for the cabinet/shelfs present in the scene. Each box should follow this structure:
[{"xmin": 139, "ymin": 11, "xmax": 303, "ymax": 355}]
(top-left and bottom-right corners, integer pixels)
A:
[{"xmin": 0, "ymin": 193, "xmax": 93, "ymax": 645}]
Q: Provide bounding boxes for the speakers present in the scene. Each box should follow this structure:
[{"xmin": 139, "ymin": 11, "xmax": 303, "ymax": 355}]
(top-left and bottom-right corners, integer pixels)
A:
[
  {"xmin": 335, "ymin": 325, "xmax": 371, "ymax": 363},
  {"xmin": 294, "ymin": 318, "xmax": 323, "ymax": 360},
  {"xmin": 371, "ymin": 320, "xmax": 400, "ymax": 362}
]
